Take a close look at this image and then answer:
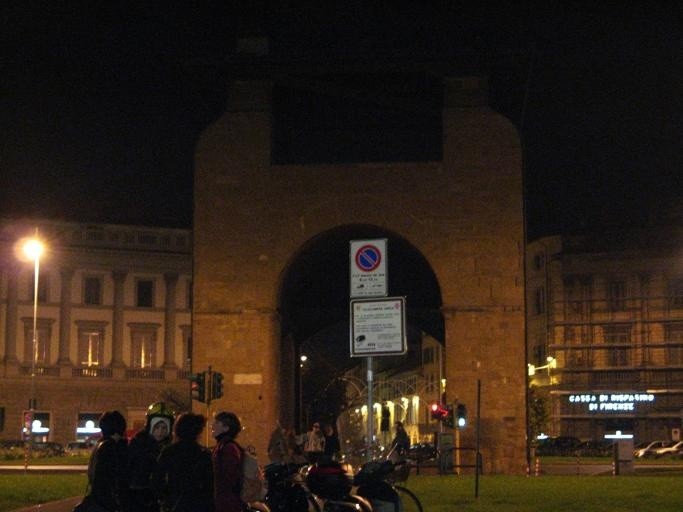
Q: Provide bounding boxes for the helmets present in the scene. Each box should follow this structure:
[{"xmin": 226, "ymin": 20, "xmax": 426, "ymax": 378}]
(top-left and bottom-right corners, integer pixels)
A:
[{"xmin": 145, "ymin": 400, "xmax": 175, "ymax": 418}]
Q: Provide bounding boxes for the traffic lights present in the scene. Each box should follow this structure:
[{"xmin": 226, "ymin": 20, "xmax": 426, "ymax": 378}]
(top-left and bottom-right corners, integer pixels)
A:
[
  {"xmin": 210, "ymin": 373, "xmax": 223, "ymax": 399},
  {"xmin": 188, "ymin": 370, "xmax": 205, "ymax": 404},
  {"xmin": 456, "ymin": 404, "xmax": 466, "ymax": 427},
  {"xmin": 431, "ymin": 406, "xmax": 449, "ymax": 424}
]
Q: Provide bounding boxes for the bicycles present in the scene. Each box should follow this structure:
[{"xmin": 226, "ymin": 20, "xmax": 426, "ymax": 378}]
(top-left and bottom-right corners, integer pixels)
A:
[{"xmin": 247, "ymin": 443, "xmax": 423, "ymax": 512}]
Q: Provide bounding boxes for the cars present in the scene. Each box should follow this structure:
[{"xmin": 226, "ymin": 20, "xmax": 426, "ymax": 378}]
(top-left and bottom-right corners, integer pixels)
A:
[
  {"xmin": 408, "ymin": 445, "xmax": 436, "ymax": 460},
  {"xmin": 633, "ymin": 439, "xmax": 683, "ymax": 458},
  {"xmin": 568, "ymin": 439, "xmax": 613, "ymax": 459}
]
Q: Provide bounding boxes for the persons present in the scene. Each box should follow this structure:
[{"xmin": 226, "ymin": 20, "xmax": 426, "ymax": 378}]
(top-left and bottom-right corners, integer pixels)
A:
[
  {"xmin": 87, "ymin": 409, "xmax": 247, "ymax": 512},
  {"xmin": 391, "ymin": 421, "xmax": 412, "ymax": 482},
  {"xmin": 268, "ymin": 421, "xmax": 342, "ymax": 466}
]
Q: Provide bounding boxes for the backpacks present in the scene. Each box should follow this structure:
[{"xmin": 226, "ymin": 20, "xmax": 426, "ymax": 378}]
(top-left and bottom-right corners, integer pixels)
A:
[{"xmin": 218, "ymin": 441, "xmax": 268, "ymax": 506}]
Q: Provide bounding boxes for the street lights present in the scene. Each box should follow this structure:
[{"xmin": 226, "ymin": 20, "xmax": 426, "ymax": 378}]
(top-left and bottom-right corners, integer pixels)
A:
[{"xmin": 20, "ymin": 226, "xmax": 41, "ymax": 468}]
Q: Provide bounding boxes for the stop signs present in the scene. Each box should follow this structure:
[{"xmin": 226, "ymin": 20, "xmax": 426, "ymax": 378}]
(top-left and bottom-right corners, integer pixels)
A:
[{"xmin": 347, "ymin": 239, "xmax": 389, "ymax": 300}]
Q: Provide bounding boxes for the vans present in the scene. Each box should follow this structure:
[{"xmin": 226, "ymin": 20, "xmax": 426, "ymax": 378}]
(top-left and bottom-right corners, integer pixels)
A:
[{"xmin": 534, "ymin": 436, "xmax": 581, "ymax": 458}]
